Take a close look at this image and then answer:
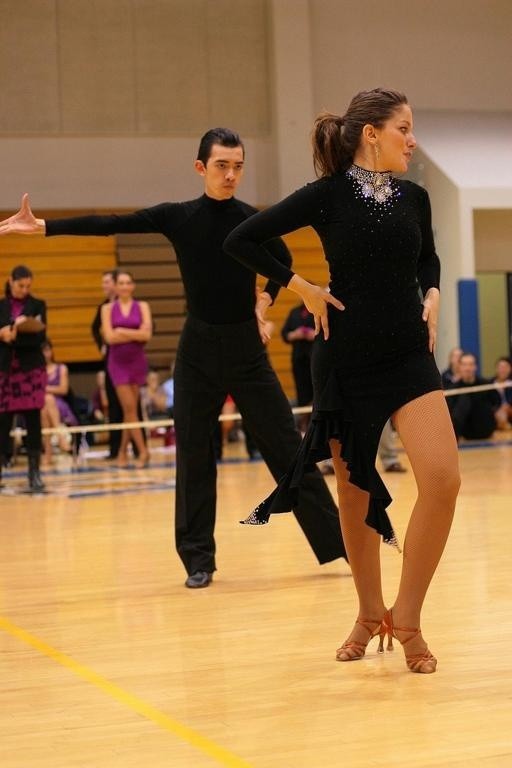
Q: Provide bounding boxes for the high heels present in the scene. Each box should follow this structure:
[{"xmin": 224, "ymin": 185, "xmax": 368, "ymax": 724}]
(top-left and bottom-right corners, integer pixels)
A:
[
  {"xmin": 338, "ymin": 610, "xmax": 388, "ymax": 661},
  {"xmin": 118, "ymin": 457, "xmax": 128, "ymax": 468},
  {"xmin": 385, "ymin": 608, "xmax": 438, "ymax": 674},
  {"xmin": 133, "ymin": 451, "xmax": 149, "ymax": 469}
]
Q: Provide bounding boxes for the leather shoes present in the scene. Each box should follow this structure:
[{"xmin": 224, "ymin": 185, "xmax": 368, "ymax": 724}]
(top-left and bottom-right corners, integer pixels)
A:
[{"xmin": 187, "ymin": 571, "xmax": 215, "ymax": 587}]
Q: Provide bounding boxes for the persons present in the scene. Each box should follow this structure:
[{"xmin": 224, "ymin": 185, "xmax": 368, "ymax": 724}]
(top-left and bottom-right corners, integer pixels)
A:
[
  {"xmin": 99, "ymin": 269, "xmax": 156, "ymax": 468},
  {"xmin": 0, "ymin": 339, "xmax": 511, "ymax": 476},
  {"xmin": 0, "ymin": 265, "xmax": 49, "ymax": 489},
  {"xmin": 89, "ymin": 270, "xmax": 149, "ymax": 460},
  {"xmin": 221, "ymin": 86, "xmax": 463, "ymax": 674},
  {"xmin": 280, "ymin": 279, "xmax": 323, "ymax": 405},
  {"xmin": 1, "ymin": 126, "xmax": 352, "ymax": 589}
]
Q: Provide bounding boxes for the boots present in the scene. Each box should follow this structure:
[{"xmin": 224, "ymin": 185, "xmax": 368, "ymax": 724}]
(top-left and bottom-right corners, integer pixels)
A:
[{"xmin": 28, "ymin": 442, "xmax": 44, "ymax": 489}]
